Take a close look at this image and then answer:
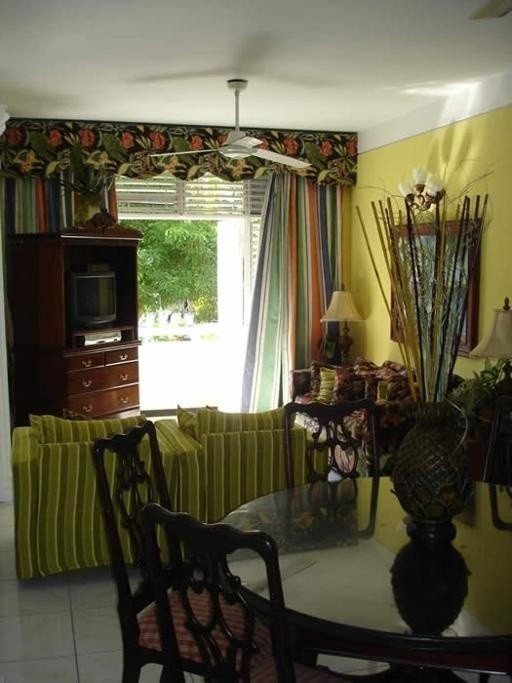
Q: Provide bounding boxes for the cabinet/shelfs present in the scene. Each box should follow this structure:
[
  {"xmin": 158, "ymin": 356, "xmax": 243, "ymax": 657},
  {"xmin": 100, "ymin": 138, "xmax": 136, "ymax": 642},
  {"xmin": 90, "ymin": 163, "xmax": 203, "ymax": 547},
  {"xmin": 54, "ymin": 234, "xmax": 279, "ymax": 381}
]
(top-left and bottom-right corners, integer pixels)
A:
[
  {"xmin": 10, "ymin": 345, "xmax": 140, "ymax": 430},
  {"xmin": 6, "ymin": 206, "xmax": 143, "ymax": 356}
]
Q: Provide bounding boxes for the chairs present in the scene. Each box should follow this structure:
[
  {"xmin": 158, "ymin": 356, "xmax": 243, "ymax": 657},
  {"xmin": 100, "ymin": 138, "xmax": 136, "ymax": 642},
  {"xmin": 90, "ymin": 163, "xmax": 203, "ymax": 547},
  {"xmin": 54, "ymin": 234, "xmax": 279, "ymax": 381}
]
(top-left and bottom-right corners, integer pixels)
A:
[
  {"xmin": 284, "ymin": 400, "xmax": 378, "ymax": 483},
  {"xmin": 287, "ymin": 476, "xmax": 379, "ymax": 548},
  {"xmin": 127, "ymin": 501, "xmax": 340, "ymax": 682},
  {"xmin": 93, "ymin": 419, "xmax": 273, "ymax": 683},
  {"xmin": 11, "ymin": 406, "xmax": 175, "ymax": 579},
  {"xmin": 154, "ymin": 401, "xmax": 326, "ymax": 550}
]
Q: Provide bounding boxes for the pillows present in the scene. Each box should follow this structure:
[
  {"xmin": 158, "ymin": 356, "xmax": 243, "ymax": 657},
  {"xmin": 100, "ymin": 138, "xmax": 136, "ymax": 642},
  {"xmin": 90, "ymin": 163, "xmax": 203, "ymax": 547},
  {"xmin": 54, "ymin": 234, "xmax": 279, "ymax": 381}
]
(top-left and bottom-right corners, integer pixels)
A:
[
  {"xmin": 24, "ymin": 411, "xmax": 144, "ymax": 440},
  {"xmin": 174, "ymin": 401, "xmax": 199, "ymax": 435},
  {"xmin": 193, "ymin": 403, "xmax": 297, "ymax": 432},
  {"xmin": 306, "ymin": 361, "xmax": 411, "ymax": 402}
]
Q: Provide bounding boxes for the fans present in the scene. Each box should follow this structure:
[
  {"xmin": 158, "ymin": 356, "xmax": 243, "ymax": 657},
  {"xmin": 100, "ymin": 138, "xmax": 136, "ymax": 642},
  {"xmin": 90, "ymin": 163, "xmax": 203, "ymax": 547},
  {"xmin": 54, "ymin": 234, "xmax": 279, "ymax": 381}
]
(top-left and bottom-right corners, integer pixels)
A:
[{"xmin": 146, "ymin": 70, "xmax": 319, "ymax": 169}]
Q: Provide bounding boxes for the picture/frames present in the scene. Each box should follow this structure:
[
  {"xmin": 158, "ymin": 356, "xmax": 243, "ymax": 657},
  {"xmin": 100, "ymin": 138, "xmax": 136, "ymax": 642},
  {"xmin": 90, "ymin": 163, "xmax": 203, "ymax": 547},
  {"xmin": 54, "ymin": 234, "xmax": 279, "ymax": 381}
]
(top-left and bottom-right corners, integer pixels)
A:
[{"xmin": 390, "ymin": 216, "xmax": 483, "ymax": 358}]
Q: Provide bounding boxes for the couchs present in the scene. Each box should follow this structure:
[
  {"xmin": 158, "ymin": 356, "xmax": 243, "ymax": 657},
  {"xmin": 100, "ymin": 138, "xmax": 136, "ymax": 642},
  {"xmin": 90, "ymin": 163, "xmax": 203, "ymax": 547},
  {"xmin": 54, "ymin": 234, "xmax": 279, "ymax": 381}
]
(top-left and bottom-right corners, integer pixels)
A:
[{"xmin": 288, "ymin": 359, "xmax": 464, "ymax": 462}]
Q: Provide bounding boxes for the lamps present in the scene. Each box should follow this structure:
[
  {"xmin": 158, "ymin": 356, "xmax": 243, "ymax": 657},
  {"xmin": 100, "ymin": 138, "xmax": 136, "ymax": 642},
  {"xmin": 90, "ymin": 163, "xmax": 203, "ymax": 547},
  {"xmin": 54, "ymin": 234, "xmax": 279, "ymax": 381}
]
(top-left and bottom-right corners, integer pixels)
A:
[
  {"xmin": 468, "ymin": 295, "xmax": 511, "ymax": 398},
  {"xmin": 318, "ymin": 282, "xmax": 365, "ymax": 360},
  {"xmin": 399, "ymin": 165, "xmax": 446, "ymax": 206}
]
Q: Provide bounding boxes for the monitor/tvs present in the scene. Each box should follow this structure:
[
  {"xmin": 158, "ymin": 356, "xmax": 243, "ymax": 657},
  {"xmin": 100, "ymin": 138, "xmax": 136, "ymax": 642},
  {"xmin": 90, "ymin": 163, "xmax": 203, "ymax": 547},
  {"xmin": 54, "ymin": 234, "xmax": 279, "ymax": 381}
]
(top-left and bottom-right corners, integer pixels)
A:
[{"xmin": 65, "ymin": 271, "xmax": 117, "ymax": 327}]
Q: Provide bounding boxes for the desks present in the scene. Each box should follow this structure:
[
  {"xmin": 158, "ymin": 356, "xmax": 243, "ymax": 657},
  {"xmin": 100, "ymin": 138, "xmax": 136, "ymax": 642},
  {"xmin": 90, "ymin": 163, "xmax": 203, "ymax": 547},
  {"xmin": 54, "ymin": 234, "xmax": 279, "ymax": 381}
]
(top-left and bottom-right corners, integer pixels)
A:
[{"xmin": 215, "ymin": 470, "xmax": 511, "ymax": 682}]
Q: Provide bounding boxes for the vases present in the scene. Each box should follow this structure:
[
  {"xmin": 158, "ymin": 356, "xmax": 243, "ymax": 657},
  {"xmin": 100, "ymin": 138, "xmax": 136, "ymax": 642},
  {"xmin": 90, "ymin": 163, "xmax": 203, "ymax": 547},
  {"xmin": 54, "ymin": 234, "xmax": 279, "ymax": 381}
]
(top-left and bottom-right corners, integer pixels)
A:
[
  {"xmin": 389, "ymin": 402, "xmax": 470, "ymax": 528},
  {"xmin": 389, "ymin": 528, "xmax": 469, "ymax": 635}
]
